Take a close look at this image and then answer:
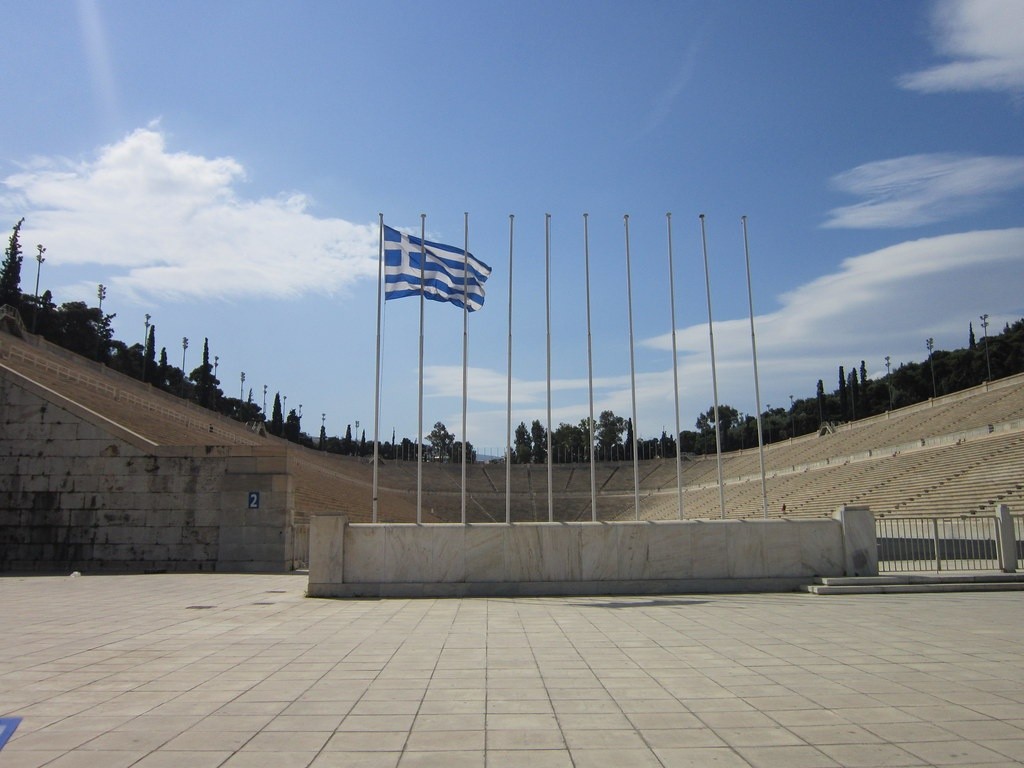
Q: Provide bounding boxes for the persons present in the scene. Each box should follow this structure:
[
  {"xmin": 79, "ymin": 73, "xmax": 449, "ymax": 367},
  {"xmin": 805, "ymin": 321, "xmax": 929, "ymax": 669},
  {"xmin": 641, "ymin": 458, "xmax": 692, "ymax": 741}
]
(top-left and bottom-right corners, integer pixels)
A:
[
  {"xmin": 783, "ymin": 503, "xmax": 786, "ymax": 512},
  {"xmin": 210, "ymin": 424, "xmax": 213, "ymax": 432}
]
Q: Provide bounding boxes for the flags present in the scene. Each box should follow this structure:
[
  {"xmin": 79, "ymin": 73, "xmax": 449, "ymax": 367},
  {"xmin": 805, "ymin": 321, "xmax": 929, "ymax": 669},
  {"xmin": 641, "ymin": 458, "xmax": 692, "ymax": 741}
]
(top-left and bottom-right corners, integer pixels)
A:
[{"xmin": 383, "ymin": 226, "xmax": 491, "ymax": 312}]
